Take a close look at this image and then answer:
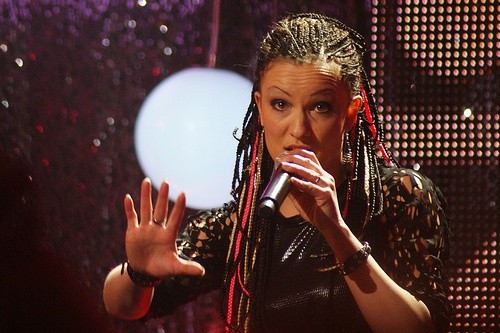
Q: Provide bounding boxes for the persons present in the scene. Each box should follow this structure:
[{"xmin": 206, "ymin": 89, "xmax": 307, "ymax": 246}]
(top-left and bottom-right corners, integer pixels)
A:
[{"xmin": 103, "ymin": 13, "xmax": 453, "ymax": 333}]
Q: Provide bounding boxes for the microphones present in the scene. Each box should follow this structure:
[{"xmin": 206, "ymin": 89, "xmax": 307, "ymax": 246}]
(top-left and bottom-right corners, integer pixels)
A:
[{"xmin": 258, "ymin": 161, "xmax": 299, "ymax": 219}]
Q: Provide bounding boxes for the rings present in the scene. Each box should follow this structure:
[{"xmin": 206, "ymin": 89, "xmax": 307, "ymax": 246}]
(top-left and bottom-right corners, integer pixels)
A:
[
  {"xmin": 153, "ymin": 216, "xmax": 167, "ymax": 224},
  {"xmin": 315, "ymin": 173, "xmax": 322, "ymax": 183}
]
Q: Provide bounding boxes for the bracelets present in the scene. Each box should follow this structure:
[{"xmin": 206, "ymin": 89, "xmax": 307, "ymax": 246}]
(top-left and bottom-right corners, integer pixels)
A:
[
  {"xmin": 127, "ymin": 262, "xmax": 161, "ymax": 286},
  {"xmin": 336, "ymin": 241, "xmax": 372, "ymax": 276}
]
